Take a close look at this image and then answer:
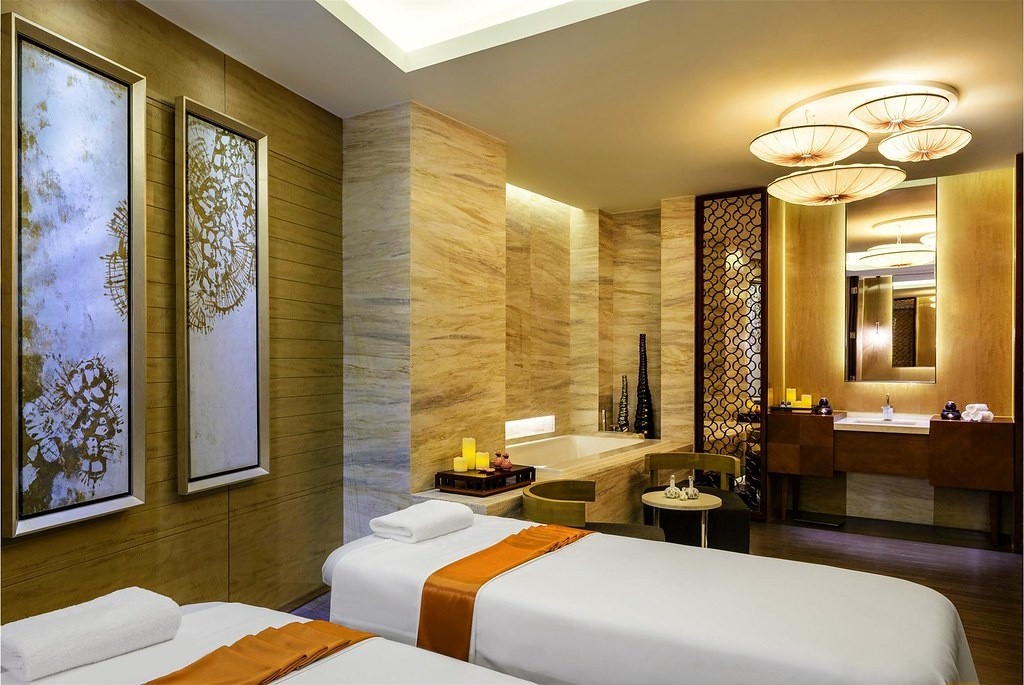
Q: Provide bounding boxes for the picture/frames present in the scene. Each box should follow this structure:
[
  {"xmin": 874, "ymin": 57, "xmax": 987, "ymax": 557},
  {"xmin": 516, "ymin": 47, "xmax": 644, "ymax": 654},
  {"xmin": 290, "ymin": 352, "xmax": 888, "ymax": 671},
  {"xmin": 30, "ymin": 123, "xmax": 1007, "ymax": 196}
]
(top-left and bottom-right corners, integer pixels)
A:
[
  {"xmin": 174, "ymin": 94, "xmax": 274, "ymax": 498},
  {"xmin": 0, "ymin": 11, "xmax": 146, "ymax": 541}
]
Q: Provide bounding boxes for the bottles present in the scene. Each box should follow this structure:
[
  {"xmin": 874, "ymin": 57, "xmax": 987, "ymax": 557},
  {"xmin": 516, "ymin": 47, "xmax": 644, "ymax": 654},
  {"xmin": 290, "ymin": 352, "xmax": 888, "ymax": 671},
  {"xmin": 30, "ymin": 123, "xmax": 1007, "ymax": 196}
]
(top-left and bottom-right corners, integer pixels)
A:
[
  {"xmin": 685, "ymin": 476, "xmax": 699, "ymax": 499},
  {"xmin": 664, "ymin": 475, "xmax": 679, "ymax": 499}
]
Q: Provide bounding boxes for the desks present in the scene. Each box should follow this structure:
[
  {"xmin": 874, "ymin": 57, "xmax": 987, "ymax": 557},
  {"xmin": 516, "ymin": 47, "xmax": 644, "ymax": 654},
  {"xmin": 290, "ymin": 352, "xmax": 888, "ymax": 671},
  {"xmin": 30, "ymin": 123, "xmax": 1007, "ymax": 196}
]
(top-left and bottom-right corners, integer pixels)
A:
[{"xmin": 639, "ymin": 490, "xmax": 721, "ymax": 548}]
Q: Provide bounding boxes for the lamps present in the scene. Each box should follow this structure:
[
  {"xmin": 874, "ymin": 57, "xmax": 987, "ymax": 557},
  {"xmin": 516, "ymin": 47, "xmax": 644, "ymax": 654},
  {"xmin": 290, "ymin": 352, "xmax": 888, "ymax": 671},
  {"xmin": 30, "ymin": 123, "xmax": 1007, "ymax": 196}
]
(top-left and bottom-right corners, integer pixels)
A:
[
  {"xmin": 877, "ymin": 124, "xmax": 973, "ymax": 162},
  {"xmin": 848, "ymin": 88, "xmax": 950, "ymax": 134},
  {"xmin": 858, "ymin": 225, "xmax": 936, "ymax": 268},
  {"xmin": 920, "ymin": 232, "xmax": 936, "ymax": 247},
  {"xmin": 867, "ymin": 242, "xmax": 924, "ymax": 253},
  {"xmin": 747, "ymin": 106, "xmax": 868, "ymax": 167},
  {"xmin": 766, "ymin": 161, "xmax": 907, "ymax": 206}
]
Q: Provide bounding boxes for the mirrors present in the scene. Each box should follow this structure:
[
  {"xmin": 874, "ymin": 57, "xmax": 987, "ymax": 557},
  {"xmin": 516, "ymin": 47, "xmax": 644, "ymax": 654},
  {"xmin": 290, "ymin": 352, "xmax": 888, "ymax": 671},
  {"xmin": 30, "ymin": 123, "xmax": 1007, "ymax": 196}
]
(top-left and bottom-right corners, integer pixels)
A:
[{"xmin": 844, "ymin": 183, "xmax": 937, "ymax": 384}]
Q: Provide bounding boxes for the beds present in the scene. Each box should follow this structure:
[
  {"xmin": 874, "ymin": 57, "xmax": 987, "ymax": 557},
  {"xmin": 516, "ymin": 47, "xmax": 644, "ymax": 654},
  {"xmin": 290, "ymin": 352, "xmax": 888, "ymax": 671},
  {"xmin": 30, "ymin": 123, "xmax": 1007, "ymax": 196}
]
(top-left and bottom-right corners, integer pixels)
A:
[
  {"xmin": 0, "ymin": 586, "xmax": 537, "ymax": 685},
  {"xmin": 321, "ymin": 500, "xmax": 980, "ymax": 685}
]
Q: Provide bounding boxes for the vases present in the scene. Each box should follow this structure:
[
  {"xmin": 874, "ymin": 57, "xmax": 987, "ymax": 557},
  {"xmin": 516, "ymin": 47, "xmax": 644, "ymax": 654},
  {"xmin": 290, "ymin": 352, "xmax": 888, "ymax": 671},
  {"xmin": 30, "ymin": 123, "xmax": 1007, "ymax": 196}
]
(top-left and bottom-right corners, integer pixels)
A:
[
  {"xmin": 614, "ymin": 375, "xmax": 630, "ymax": 432},
  {"xmin": 634, "ymin": 334, "xmax": 654, "ymax": 437}
]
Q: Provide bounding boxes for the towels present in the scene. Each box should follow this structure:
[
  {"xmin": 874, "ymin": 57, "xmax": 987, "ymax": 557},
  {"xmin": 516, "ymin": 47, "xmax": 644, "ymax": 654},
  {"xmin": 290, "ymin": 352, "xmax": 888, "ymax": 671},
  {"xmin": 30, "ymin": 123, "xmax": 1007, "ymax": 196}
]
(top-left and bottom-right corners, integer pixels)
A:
[
  {"xmin": 369, "ymin": 499, "xmax": 474, "ymax": 545},
  {"xmin": 1, "ymin": 585, "xmax": 182, "ymax": 682},
  {"xmin": 961, "ymin": 403, "xmax": 994, "ymax": 421}
]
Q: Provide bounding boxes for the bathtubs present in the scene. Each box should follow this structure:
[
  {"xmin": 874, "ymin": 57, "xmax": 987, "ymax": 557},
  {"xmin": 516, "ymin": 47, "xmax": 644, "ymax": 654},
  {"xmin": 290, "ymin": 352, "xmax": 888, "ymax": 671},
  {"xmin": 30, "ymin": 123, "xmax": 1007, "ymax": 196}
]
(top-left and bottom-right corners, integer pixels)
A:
[{"xmin": 505, "ymin": 436, "xmax": 646, "ymax": 469}]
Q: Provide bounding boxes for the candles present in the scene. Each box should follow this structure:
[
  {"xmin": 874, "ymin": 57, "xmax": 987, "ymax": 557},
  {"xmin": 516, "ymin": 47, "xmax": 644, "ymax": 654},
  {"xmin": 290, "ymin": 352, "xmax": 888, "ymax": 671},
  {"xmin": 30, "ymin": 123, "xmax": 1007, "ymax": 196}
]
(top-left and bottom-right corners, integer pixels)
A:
[
  {"xmin": 475, "ymin": 452, "xmax": 490, "ymax": 470},
  {"xmin": 453, "ymin": 456, "xmax": 467, "ymax": 472},
  {"xmin": 462, "ymin": 437, "xmax": 476, "ymax": 469}
]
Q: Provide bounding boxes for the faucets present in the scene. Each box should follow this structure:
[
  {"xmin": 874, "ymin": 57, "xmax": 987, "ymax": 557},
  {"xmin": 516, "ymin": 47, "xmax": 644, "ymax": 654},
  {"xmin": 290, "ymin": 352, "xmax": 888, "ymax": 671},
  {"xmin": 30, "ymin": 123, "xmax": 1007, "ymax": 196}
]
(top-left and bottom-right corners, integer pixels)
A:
[{"xmin": 881, "ymin": 392, "xmax": 894, "ymax": 420}]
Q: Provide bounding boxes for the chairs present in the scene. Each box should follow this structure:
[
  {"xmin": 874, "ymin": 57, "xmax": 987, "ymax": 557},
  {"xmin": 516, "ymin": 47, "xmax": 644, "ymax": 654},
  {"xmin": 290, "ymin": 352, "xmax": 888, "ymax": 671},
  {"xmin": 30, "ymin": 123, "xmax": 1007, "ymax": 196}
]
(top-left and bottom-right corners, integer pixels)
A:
[
  {"xmin": 643, "ymin": 452, "xmax": 751, "ymax": 553},
  {"xmin": 522, "ymin": 480, "xmax": 667, "ymax": 543}
]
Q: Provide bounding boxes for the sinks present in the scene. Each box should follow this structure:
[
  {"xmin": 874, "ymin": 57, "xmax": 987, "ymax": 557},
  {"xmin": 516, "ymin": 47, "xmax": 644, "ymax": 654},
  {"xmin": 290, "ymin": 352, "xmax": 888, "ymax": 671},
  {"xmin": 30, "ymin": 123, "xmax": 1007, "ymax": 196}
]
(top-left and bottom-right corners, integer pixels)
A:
[{"xmin": 836, "ymin": 416, "xmax": 930, "ymax": 427}]
